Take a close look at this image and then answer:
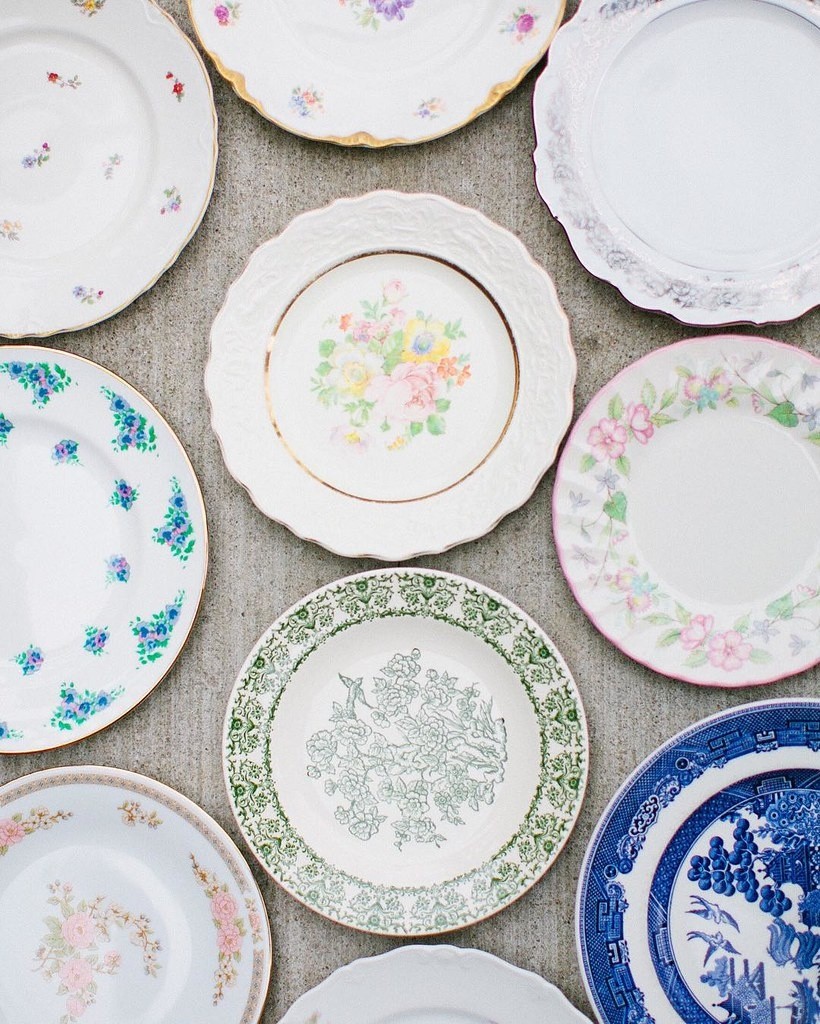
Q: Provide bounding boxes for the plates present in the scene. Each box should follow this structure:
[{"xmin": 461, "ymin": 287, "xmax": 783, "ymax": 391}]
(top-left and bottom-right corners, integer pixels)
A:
[
  {"xmin": 0, "ymin": 344, "xmax": 208, "ymax": 754},
  {"xmin": 275, "ymin": 945, "xmax": 594, "ymax": 1024},
  {"xmin": 552, "ymin": 335, "xmax": 820, "ymax": 686},
  {"xmin": 0, "ymin": 0, "xmax": 219, "ymax": 339},
  {"xmin": 222, "ymin": 567, "xmax": 590, "ymax": 935},
  {"xmin": 202, "ymin": 188, "xmax": 576, "ymax": 562},
  {"xmin": 0, "ymin": 764, "xmax": 271, "ymax": 1024},
  {"xmin": 185, "ymin": 0, "xmax": 564, "ymax": 149},
  {"xmin": 575, "ymin": 699, "xmax": 820, "ymax": 1024},
  {"xmin": 532, "ymin": 0, "xmax": 820, "ymax": 328}
]
